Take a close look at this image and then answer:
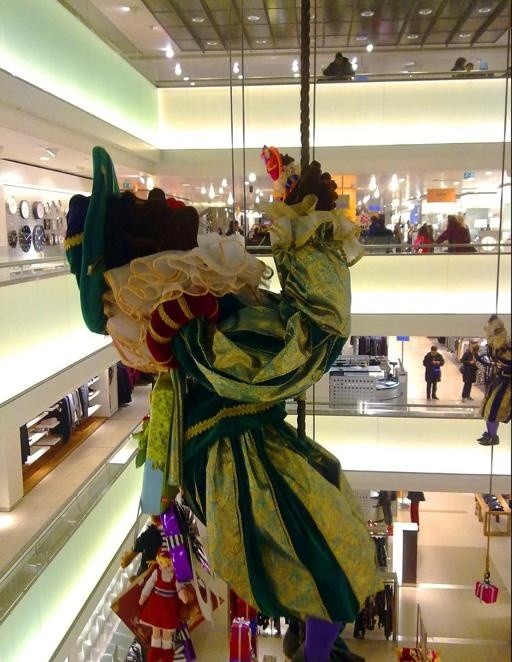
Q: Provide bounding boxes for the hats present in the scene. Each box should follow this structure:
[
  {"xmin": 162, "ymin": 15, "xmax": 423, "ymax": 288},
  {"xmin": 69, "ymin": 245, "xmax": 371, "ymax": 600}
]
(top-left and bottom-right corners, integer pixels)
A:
[{"xmin": 66, "ymin": 145, "xmax": 122, "ymax": 333}]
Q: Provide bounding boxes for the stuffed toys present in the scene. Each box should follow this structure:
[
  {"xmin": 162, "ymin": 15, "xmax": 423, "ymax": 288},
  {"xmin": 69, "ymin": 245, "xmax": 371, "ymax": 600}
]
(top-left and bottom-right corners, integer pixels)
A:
[{"xmin": 136, "ymin": 549, "xmax": 194, "ymax": 661}]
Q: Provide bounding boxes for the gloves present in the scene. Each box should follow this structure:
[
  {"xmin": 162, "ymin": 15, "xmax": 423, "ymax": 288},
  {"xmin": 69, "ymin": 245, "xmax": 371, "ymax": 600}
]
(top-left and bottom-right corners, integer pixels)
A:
[{"xmin": 283, "ymin": 160, "xmax": 338, "ymax": 211}]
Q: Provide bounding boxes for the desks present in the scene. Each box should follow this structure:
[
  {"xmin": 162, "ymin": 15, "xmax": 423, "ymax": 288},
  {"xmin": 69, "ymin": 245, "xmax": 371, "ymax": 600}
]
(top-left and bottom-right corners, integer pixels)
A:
[{"xmin": 474, "ymin": 493, "xmax": 512, "ymax": 538}]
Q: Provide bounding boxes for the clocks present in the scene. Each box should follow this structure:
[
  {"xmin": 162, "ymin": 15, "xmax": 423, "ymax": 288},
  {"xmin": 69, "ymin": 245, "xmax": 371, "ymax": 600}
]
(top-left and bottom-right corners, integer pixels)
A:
[
  {"xmin": 32, "ymin": 224, "xmax": 46, "ymax": 253},
  {"xmin": 8, "ymin": 230, "xmax": 18, "ymax": 249},
  {"xmin": 19, "ymin": 225, "xmax": 32, "ymax": 254}
]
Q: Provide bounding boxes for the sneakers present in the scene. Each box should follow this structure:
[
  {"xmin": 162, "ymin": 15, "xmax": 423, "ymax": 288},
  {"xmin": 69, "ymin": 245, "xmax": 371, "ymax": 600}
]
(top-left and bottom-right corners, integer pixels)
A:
[
  {"xmin": 477, "ymin": 432, "xmax": 499, "ymax": 445},
  {"xmin": 467, "ymin": 396, "xmax": 474, "ymax": 401},
  {"xmin": 431, "ymin": 395, "xmax": 438, "ymax": 400},
  {"xmin": 461, "ymin": 397, "xmax": 466, "ymax": 403},
  {"xmin": 426, "ymin": 396, "xmax": 430, "ymax": 400}
]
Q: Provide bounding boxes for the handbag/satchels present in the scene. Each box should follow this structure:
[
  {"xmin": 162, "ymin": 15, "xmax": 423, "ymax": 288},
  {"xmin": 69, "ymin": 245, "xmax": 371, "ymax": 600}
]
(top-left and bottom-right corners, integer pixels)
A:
[
  {"xmin": 433, "ymin": 366, "xmax": 440, "ymax": 381},
  {"xmin": 460, "ymin": 365, "xmax": 478, "ymax": 373}
]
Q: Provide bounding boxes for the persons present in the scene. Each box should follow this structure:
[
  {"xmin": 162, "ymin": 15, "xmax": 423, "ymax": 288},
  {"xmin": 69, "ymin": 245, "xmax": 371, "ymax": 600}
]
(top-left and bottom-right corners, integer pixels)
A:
[
  {"xmin": 450, "ymin": 57, "xmax": 466, "ymax": 78},
  {"xmin": 477, "ymin": 314, "xmax": 511, "ymax": 446},
  {"xmin": 65, "ymin": 145, "xmax": 377, "ymax": 662},
  {"xmin": 226, "ymin": 213, "xmax": 477, "ymax": 253},
  {"xmin": 458, "ymin": 343, "xmax": 480, "ymax": 402},
  {"xmin": 466, "ymin": 62, "xmax": 476, "ymax": 77},
  {"xmin": 423, "ymin": 345, "xmax": 445, "ymax": 400},
  {"xmin": 322, "ymin": 51, "xmax": 356, "ymax": 80}
]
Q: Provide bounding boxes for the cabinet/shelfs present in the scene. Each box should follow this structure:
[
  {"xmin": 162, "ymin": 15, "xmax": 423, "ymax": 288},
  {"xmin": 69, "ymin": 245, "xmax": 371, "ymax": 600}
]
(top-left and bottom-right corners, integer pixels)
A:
[{"xmin": 23, "ymin": 376, "xmax": 102, "ymax": 468}]
